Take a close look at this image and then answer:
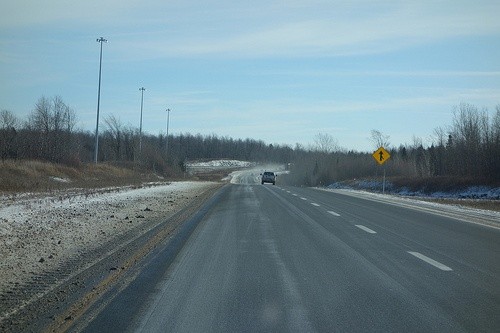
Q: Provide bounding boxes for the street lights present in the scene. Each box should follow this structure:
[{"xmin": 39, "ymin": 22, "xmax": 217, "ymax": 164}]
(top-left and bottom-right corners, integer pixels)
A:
[
  {"xmin": 138, "ymin": 86, "xmax": 145, "ymax": 152},
  {"xmin": 165, "ymin": 109, "xmax": 171, "ymax": 150},
  {"xmin": 93, "ymin": 35, "xmax": 107, "ymax": 163}
]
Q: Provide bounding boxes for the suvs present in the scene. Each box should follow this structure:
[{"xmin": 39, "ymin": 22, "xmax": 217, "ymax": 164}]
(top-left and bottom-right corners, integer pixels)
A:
[{"xmin": 260, "ymin": 172, "xmax": 277, "ymax": 185}]
